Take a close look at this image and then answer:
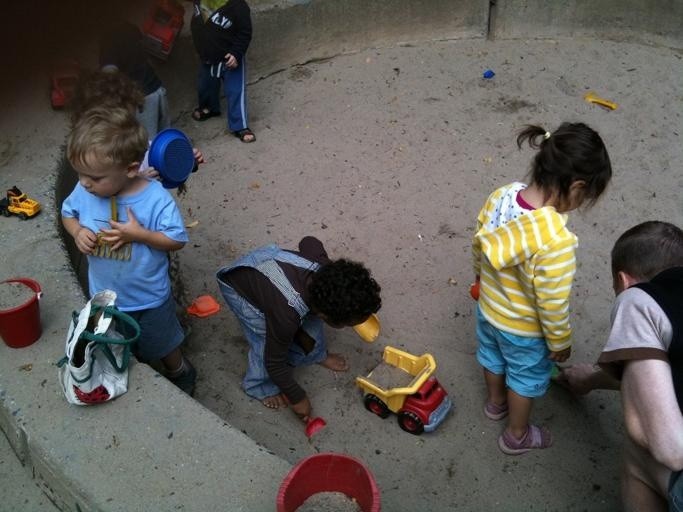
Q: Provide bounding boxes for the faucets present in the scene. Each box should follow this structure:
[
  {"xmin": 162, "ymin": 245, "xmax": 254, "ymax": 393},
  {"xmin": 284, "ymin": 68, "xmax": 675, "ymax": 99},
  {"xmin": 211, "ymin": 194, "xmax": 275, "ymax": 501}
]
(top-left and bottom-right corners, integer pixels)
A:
[
  {"xmin": 483, "ymin": 398, "xmax": 509, "ymax": 420},
  {"xmin": 498, "ymin": 423, "xmax": 554, "ymax": 455},
  {"xmin": 191, "ymin": 106, "xmax": 220, "ymax": 121},
  {"xmin": 234, "ymin": 128, "xmax": 255, "ymax": 143}
]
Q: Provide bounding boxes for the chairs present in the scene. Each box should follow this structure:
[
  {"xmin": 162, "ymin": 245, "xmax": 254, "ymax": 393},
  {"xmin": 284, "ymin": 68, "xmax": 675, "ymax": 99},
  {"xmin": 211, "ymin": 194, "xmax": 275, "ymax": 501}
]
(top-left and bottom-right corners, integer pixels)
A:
[{"xmin": 167, "ymin": 356, "xmax": 196, "ymax": 398}]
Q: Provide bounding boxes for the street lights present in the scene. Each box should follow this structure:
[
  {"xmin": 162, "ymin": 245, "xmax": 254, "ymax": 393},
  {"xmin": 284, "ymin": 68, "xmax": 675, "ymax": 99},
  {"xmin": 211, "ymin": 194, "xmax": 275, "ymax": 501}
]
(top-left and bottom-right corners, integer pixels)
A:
[{"xmin": 57, "ymin": 289, "xmax": 127, "ymax": 406}]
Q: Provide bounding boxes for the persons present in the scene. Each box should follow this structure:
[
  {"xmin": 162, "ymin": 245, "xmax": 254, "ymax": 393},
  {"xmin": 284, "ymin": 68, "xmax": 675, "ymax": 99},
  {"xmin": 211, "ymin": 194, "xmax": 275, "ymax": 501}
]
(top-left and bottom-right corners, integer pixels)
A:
[
  {"xmin": 59, "ymin": 71, "xmax": 204, "ymax": 254},
  {"xmin": 98, "ymin": 20, "xmax": 171, "ymax": 144},
  {"xmin": 215, "ymin": 236, "xmax": 380, "ymax": 422},
  {"xmin": 190, "ymin": 1, "xmax": 256, "ymax": 143},
  {"xmin": 556, "ymin": 221, "xmax": 682, "ymax": 512},
  {"xmin": 470, "ymin": 122, "xmax": 612, "ymax": 456},
  {"xmin": 61, "ymin": 104, "xmax": 197, "ymax": 398}
]
